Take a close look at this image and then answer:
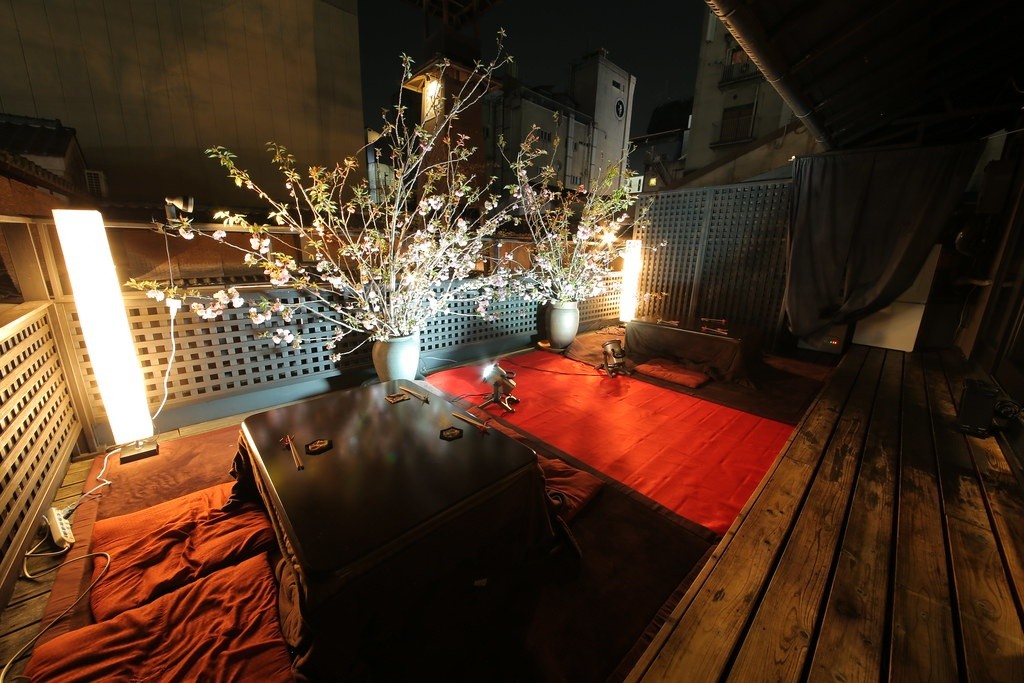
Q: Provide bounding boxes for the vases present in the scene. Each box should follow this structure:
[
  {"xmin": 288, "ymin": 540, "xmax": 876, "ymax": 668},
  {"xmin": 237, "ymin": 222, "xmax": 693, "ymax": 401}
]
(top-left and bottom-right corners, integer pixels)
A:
[
  {"xmin": 543, "ymin": 297, "xmax": 579, "ymax": 347},
  {"xmin": 370, "ymin": 332, "xmax": 421, "ymax": 384}
]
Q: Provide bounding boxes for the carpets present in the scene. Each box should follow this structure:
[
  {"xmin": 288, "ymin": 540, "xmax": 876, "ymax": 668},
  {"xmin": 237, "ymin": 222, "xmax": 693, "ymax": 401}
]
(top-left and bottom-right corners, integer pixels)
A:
[
  {"xmin": 563, "ymin": 328, "xmax": 822, "ymax": 424},
  {"xmin": 17, "ymin": 378, "xmax": 721, "ymax": 682}
]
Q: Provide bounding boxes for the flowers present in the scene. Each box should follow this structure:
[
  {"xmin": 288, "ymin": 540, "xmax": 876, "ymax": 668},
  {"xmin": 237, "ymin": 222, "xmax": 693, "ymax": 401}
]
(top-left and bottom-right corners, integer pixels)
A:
[
  {"xmin": 496, "ymin": 112, "xmax": 669, "ymax": 306},
  {"xmin": 167, "ymin": 26, "xmax": 560, "ymax": 363}
]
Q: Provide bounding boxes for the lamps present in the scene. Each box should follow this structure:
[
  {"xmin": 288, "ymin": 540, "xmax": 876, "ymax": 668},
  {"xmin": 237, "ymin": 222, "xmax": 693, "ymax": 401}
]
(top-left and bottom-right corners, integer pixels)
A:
[
  {"xmin": 594, "ymin": 339, "xmax": 631, "ymax": 378},
  {"xmin": 477, "ymin": 359, "xmax": 520, "ymax": 414}
]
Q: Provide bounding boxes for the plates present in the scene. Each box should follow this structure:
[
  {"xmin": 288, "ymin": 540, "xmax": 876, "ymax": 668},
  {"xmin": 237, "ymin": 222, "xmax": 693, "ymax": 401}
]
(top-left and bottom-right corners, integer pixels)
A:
[
  {"xmin": 440, "ymin": 427, "xmax": 463, "ymax": 441},
  {"xmin": 305, "ymin": 439, "xmax": 333, "ymax": 455},
  {"xmin": 385, "ymin": 393, "xmax": 409, "ymax": 403}
]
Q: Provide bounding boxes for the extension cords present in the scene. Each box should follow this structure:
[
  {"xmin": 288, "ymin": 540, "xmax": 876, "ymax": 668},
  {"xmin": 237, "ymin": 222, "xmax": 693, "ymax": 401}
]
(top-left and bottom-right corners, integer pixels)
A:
[
  {"xmin": 166, "ymin": 298, "xmax": 181, "ymax": 309},
  {"xmin": 46, "ymin": 507, "xmax": 76, "ymax": 549}
]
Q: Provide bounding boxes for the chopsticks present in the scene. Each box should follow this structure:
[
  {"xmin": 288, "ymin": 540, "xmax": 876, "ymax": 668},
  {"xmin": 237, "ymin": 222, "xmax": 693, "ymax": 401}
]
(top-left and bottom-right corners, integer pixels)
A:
[
  {"xmin": 452, "ymin": 411, "xmax": 485, "ymax": 429},
  {"xmin": 400, "ymin": 387, "xmax": 424, "ymax": 401},
  {"xmin": 287, "ymin": 435, "xmax": 304, "ymax": 470}
]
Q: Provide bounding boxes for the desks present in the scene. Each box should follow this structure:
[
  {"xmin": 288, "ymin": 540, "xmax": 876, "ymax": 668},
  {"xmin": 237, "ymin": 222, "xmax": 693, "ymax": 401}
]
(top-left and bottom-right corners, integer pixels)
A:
[
  {"xmin": 220, "ymin": 377, "xmax": 586, "ymax": 683},
  {"xmin": 623, "ymin": 312, "xmax": 763, "ymax": 382}
]
